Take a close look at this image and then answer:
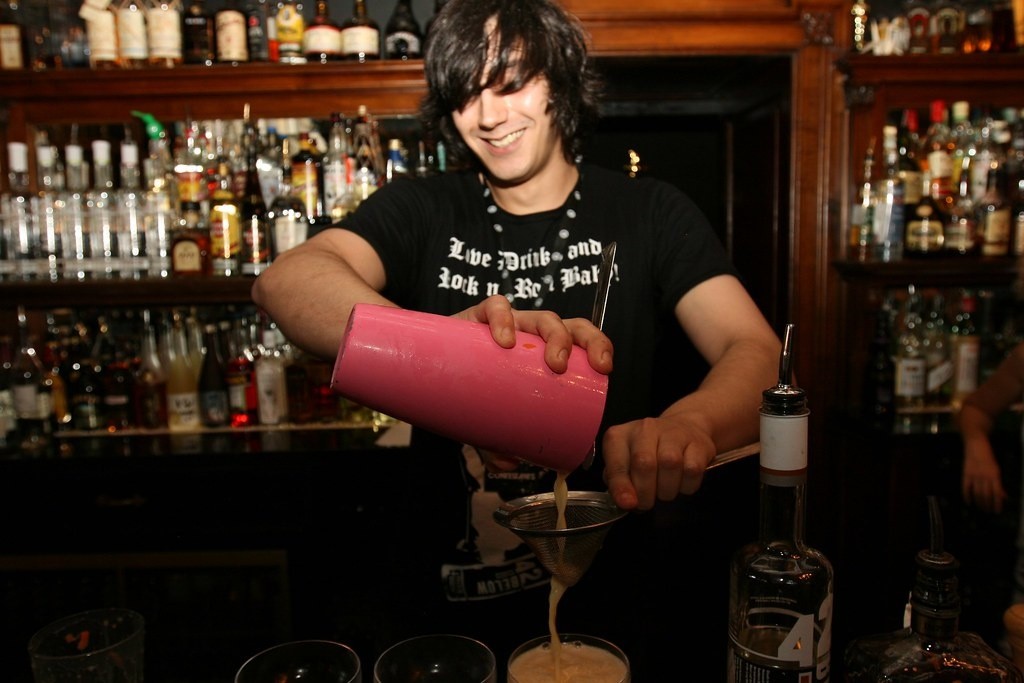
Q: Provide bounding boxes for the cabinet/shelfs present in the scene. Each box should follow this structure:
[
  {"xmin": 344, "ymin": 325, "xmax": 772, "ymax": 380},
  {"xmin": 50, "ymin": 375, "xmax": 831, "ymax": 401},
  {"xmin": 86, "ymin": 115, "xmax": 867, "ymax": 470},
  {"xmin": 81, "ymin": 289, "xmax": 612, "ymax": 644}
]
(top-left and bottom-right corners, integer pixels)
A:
[
  {"xmin": 832, "ymin": 48, "xmax": 1024, "ymax": 416},
  {"xmin": 0, "ymin": 58, "xmax": 453, "ymax": 457}
]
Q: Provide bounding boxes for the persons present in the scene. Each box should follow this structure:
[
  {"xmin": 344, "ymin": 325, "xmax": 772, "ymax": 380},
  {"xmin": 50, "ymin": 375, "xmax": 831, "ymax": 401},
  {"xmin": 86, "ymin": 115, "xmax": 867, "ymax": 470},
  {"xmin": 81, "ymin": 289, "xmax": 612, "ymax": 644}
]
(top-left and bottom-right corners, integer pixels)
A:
[
  {"xmin": 959, "ymin": 255, "xmax": 1024, "ymax": 606},
  {"xmin": 249, "ymin": 0, "xmax": 797, "ymax": 623}
]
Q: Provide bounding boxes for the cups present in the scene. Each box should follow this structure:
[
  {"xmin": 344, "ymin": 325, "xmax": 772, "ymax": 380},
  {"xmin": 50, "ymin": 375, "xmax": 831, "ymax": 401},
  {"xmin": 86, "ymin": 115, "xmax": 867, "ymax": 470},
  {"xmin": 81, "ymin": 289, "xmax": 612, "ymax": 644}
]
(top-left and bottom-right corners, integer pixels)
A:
[
  {"xmin": 330, "ymin": 303, "xmax": 608, "ymax": 474},
  {"xmin": 234, "ymin": 633, "xmax": 631, "ymax": 683},
  {"xmin": 29, "ymin": 608, "xmax": 146, "ymax": 683}
]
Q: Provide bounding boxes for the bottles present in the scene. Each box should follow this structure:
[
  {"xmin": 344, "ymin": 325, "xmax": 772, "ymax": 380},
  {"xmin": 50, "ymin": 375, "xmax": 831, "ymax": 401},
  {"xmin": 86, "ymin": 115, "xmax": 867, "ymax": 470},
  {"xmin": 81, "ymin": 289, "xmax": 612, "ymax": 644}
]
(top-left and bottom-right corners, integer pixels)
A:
[
  {"xmin": 895, "ymin": 289, "xmax": 1024, "ymax": 412},
  {"xmin": 849, "ymin": 99, "xmax": 1024, "ymax": 262},
  {"xmin": 0, "ymin": 0, "xmax": 422, "ymax": 70},
  {"xmin": 0, "ymin": 105, "xmax": 430, "ymax": 279},
  {"xmin": 843, "ymin": 551, "xmax": 1024, "ymax": 683},
  {"xmin": 729, "ymin": 390, "xmax": 833, "ymax": 683},
  {"xmin": 0, "ymin": 305, "xmax": 337, "ymax": 431}
]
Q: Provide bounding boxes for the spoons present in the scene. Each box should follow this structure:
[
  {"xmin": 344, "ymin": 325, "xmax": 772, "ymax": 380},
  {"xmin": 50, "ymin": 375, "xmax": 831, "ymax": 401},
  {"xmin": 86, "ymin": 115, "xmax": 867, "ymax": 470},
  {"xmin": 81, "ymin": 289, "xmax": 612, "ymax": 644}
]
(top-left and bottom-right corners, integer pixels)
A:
[{"xmin": 567, "ymin": 241, "xmax": 617, "ymax": 481}]
